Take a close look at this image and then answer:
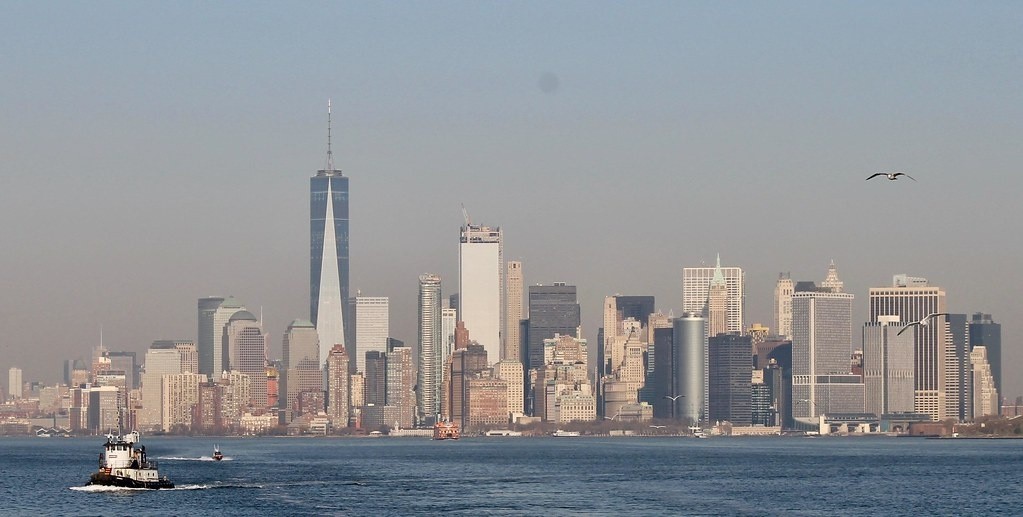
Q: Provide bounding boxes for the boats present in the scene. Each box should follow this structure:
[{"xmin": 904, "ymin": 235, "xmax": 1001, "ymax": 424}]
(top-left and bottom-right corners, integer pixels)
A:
[
  {"xmin": 433, "ymin": 421, "xmax": 459, "ymax": 440},
  {"xmin": 85, "ymin": 425, "xmax": 175, "ymax": 490},
  {"xmin": 212, "ymin": 443, "xmax": 224, "ymax": 461}
]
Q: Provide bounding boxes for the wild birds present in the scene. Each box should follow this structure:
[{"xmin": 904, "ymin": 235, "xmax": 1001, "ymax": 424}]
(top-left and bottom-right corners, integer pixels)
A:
[
  {"xmin": 603, "ymin": 414, "xmax": 619, "ymax": 421},
  {"xmin": 649, "ymin": 425, "xmax": 667, "ymax": 429},
  {"xmin": 896, "ymin": 312, "xmax": 950, "ymax": 336},
  {"xmin": 663, "ymin": 395, "xmax": 688, "ymax": 400},
  {"xmin": 797, "ymin": 398, "xmax": 816, "ymax": 406},
  {"xmin": 864, "ymin": 170, "xmax": 917, "ymax": 184}
]
